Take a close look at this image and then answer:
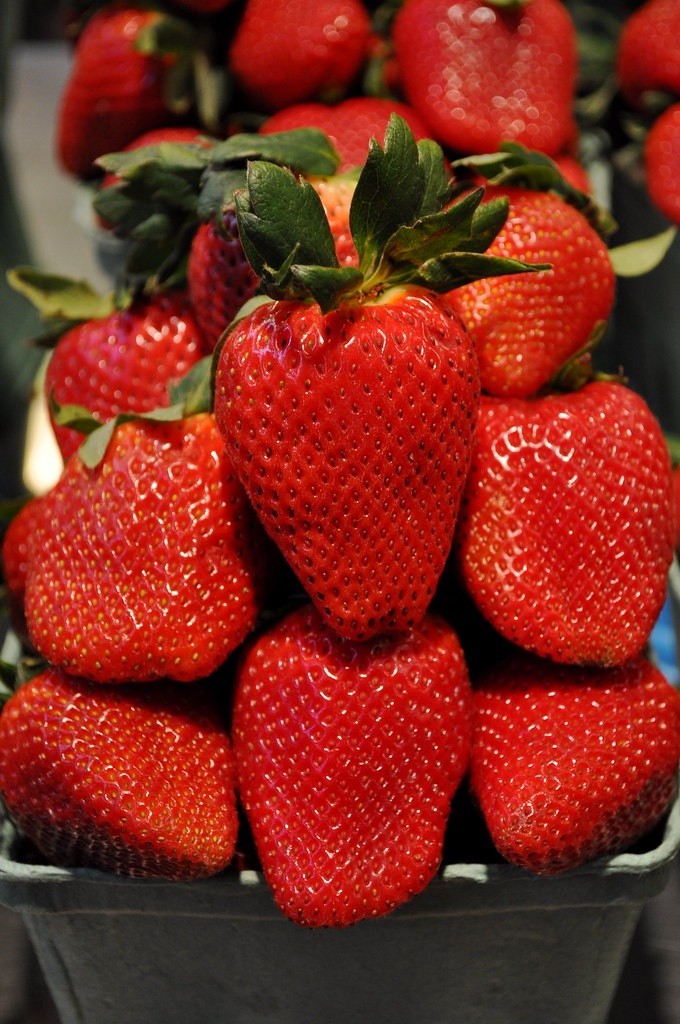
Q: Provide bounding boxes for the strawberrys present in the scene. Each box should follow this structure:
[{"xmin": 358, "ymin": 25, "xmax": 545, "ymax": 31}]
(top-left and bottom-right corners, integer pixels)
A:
[{"xmin": 1, "ymin": 0, "xmax": 680, "ymax": 926}]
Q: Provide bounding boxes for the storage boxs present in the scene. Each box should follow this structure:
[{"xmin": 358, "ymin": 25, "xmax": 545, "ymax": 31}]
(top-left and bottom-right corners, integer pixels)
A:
[{"xmin": 0, "ymin": 797, "xmax": 680, "ymax": 1024}]
